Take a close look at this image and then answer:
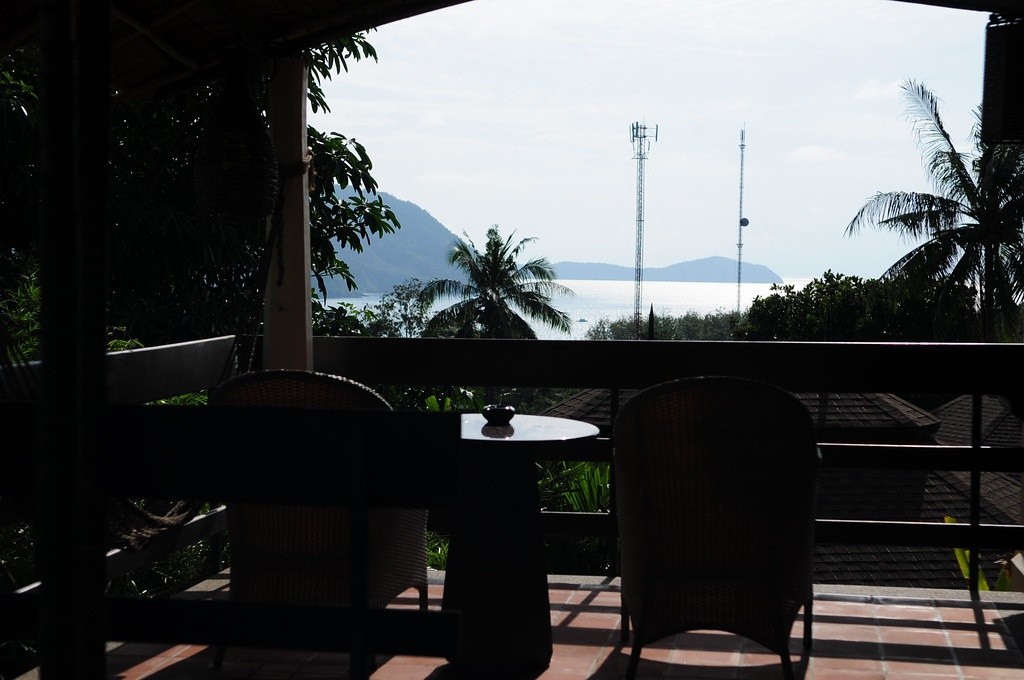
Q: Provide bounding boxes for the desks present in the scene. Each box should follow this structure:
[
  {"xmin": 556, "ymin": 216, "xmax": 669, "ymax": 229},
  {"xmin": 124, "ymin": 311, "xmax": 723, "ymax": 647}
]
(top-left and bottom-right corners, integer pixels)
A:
[{"xmin": 442, "ymin": 413, "xmax": 600, "ymax": 680}]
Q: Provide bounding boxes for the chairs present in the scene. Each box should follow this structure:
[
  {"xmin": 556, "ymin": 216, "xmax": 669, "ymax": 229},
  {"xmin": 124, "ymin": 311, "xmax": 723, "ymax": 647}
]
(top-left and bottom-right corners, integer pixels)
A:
[
  {"xmin": 208, "ymin": 370, "xmax": 428, "ymax": 680},
  {"xmin": 613, "ymin": 376, "xmax": 822, "ymax": 679}
]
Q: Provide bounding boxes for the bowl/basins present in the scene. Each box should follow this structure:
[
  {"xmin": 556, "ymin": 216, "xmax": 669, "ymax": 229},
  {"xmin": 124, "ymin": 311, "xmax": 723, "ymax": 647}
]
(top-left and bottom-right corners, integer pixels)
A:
[{"xmin": 482, "ymin": 405, "xmax": 515, "ymax": 426}]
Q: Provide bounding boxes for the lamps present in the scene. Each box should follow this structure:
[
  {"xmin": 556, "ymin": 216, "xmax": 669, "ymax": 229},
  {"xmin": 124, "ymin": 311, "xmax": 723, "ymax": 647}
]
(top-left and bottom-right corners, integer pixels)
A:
[{"xmin": 193, "ymin": 47, "xmax": 279, "ymax": 216}]
